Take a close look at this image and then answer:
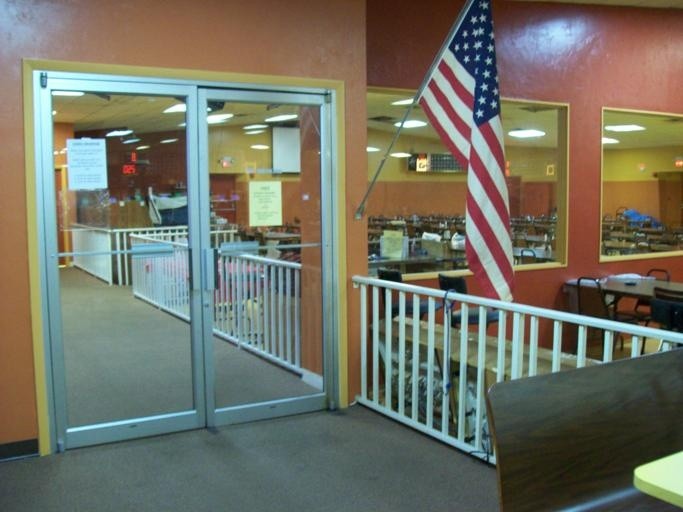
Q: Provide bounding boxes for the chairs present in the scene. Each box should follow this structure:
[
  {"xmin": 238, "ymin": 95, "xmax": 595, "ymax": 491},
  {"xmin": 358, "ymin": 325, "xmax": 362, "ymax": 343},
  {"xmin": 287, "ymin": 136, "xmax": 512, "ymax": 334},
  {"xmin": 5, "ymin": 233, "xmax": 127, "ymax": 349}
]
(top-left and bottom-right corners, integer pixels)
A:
[
  {"xmin": 651, "ymin": 286, "xmax": 683, "ymax": 354},
  {"xmin": 370, "ymin": 214, "xmax": 683, "ymax": 273},
  {"xmin": 616, "ymin": 267, "xmax": 671, "ymax": 355},
  {"xmin": 441, "ymin": 273, "xmax": 508, "ymax": 334},
  {"xmin": 377, "ymin": 270, "xmax": 443, "ymax": 324},
  {"xmin": 577, "ymin": 276, "xmax": 651, "ymax": 353},
  {"xmin": 487, "ymin": 349, "xmax": 683, "ymax": 512}
]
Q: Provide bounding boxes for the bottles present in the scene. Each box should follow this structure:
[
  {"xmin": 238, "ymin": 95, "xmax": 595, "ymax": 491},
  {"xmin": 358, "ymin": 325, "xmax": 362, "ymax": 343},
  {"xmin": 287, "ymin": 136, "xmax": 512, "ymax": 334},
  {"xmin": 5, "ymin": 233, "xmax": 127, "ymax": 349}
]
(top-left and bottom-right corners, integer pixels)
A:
[{"xmin": 410, "ymin": 238, "xmax": 417, "ymax": 256}]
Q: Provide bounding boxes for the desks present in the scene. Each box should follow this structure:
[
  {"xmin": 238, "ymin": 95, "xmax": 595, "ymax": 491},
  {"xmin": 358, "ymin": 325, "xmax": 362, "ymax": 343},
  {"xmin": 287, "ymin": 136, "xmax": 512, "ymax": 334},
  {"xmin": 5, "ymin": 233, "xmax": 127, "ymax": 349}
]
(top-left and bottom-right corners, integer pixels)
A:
[{"xmin": 634, "ymin": 451, "xmax": 683, "ymax": 512}]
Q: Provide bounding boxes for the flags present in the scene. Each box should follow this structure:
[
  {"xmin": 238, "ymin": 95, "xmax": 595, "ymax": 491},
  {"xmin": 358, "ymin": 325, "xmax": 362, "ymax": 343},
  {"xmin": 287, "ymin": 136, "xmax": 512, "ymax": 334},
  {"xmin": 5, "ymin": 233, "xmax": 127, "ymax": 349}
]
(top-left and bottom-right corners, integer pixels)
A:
[{"xmin": 418, "ymin": 1, "xmax": 515, "ymax": 307}]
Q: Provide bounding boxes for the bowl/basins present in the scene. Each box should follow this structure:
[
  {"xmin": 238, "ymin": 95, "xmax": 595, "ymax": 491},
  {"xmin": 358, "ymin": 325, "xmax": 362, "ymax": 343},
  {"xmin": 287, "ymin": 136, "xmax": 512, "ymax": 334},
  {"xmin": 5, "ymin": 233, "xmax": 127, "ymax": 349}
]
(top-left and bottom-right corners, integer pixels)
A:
[{"xmin": 534, "ymin": 247, "xmax": 546, "ymax": 255}]
